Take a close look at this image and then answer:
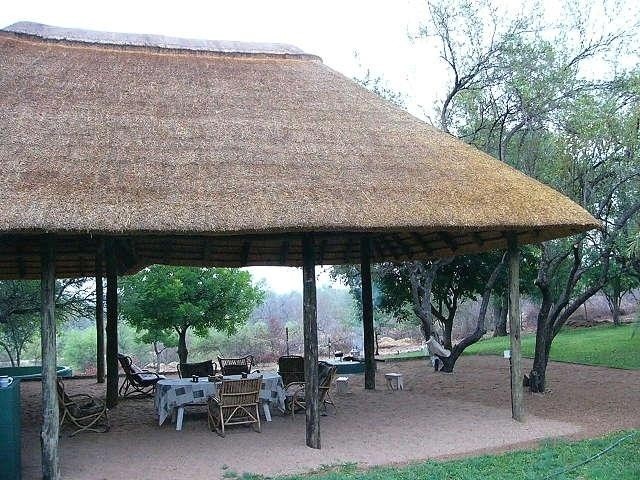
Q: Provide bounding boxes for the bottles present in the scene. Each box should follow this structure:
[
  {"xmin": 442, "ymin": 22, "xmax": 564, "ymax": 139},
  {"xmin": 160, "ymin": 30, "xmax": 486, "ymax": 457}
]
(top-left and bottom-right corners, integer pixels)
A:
[{"xmin": 192, "ymin": 375, "xmax": 199, "ymax": 382}]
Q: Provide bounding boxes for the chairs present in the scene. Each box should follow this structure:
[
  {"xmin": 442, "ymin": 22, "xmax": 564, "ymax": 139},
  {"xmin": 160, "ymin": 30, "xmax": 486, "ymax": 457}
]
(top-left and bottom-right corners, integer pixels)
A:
[
  {"xmin": 176, "ymin": 355, "xmax": 339, "ymax": 438},
  {"xmin": 57, "ymin": 376, "xmax": 110, "ymax": 437},
  {"xmin": 117, "ymin": 353, "xmax": 166, "ymax": 398}
]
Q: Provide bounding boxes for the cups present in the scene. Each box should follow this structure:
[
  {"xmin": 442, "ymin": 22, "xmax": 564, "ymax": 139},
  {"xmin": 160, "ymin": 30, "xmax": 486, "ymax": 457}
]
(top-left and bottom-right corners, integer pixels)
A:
[{"xmin": 0, "ymin": 375, "xmax": 14, "ymax": 388}]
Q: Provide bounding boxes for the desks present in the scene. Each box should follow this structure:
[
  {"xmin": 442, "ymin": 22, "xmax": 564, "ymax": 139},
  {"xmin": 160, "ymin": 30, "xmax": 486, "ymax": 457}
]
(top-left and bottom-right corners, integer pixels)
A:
[{"xmin": 157, "ymin": 373, "xmax": 283, "ymax": 431}]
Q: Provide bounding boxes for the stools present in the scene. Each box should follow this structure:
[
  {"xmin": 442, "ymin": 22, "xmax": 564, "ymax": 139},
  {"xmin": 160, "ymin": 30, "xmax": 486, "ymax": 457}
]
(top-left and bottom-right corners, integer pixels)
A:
[
  {"xmin": 335, "ymin": 376, "xmax": 349, "ymax": 392},
  {"xmin": 384, "ymin": 373, "xmax": 404, "ymax": 390}
]
